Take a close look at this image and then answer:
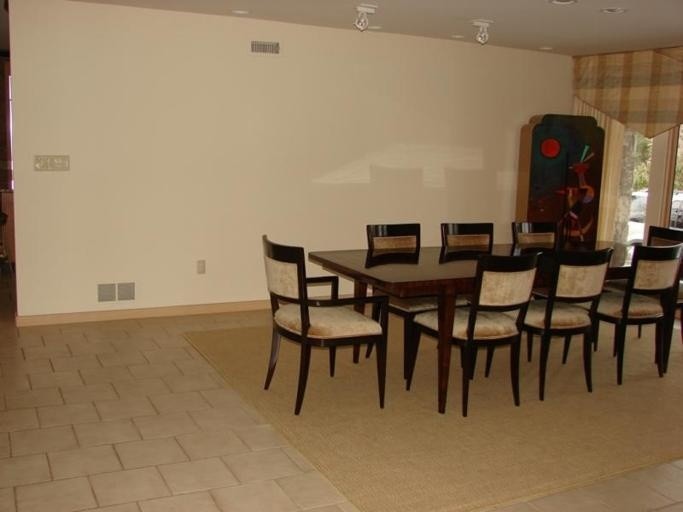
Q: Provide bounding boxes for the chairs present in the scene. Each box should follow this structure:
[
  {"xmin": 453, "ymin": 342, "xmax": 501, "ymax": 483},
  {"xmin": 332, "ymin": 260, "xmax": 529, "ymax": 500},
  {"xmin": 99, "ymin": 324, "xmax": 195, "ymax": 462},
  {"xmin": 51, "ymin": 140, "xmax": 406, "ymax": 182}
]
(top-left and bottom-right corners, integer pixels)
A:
[
  {"xmin": 365, "ymin": 223, "xmax": 469, "ymax": 379},
  {"xmin": 263, "ymin": 235, "xmax": 389, "ymax": 415},
  {"xmin": 513, "ymin": 221, "xmax": 556, "ymax": 243},
  {"xmin": 441, "ymin": 222, "xmax": 493, "ymax": 246},
  {"xmin": 563, "ymin": 243, "xmax": 683, "ymax": 385},
  {"xmin": 594, "ymin": 226, "xmax": 683, "ymax": 365},
  {"xmin": 406, "ymin": 252, "xmax": 541, "ymax": 417},
  {"xmin": 485, "ymin": 246, "xmax": 613, "ymax": 401},
  {"xmin": 0, "ymin": 211, "xmax": 13, "ymax": 278}
]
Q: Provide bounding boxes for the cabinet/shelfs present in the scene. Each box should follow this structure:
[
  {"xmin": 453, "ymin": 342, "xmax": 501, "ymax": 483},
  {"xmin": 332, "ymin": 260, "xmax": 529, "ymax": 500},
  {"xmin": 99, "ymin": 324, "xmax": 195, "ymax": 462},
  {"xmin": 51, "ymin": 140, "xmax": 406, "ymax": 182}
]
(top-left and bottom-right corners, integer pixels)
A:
[{"xmin": 516, "ymin": 114, "xmax": 605, "ymax": 241}]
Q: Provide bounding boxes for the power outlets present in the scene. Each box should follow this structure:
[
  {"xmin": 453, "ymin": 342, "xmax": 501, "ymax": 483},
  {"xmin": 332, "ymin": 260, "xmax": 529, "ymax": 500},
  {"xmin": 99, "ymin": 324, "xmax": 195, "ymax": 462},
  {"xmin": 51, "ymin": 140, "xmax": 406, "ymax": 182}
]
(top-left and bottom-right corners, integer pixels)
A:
[{"xmin": 35, "ymin": 155, "xmax": 69, "ymax": 170}]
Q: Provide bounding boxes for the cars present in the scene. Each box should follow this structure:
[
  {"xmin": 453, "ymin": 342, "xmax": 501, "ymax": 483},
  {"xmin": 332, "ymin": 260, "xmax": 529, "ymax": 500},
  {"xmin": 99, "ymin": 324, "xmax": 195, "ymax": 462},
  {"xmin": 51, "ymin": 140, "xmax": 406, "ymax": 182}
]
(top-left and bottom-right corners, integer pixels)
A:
[{"xmin": 630, "ymin": 187, "xmax": 683, "ymax": 228}]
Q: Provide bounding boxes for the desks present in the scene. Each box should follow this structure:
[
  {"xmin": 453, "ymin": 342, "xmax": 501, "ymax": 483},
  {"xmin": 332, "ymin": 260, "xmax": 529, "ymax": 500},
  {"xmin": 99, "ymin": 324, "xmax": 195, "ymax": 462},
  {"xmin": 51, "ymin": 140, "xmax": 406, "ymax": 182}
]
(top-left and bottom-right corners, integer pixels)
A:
[{"xmin": 309, "ymin": 241, "xmax": 680, "ymax": 413}]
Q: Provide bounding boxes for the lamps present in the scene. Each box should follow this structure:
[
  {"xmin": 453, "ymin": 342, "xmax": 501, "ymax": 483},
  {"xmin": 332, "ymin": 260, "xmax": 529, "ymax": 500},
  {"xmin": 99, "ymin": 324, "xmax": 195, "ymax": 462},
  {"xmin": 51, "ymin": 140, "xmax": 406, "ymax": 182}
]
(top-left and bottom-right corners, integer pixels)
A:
[
  {"xmin": 473, "ymin": 18, "xmax": 493, "ymax": 45},
  {"xmin": 353, "ymin": 4, "xmax": 377, "ymax": 31}
]
